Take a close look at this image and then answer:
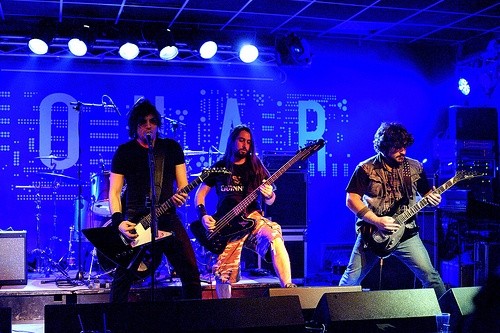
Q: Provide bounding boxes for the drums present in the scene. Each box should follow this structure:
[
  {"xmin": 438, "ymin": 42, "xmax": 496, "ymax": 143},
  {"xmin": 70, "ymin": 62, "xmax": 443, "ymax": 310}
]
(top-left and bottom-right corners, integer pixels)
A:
[
  {"xmin": 74, "ymin": 197, "xmax": 105, "ymax": 243},
  {"xmin": 95, "ymin": 217, "xmax": 164, "ymax": 281},
  {"xmin": 65, "ymin": 225, "xmax": 93, "ymax": 267},
  {"xmin": 90, "ymin": 171, "xmax": 125, "ymax": 216}
]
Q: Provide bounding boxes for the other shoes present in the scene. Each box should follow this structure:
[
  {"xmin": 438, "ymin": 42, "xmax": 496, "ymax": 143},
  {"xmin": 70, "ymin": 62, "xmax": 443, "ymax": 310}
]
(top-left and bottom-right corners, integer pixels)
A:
[{"xmin": 283, "ymin": 283, "xmax": 297, "ymax": 288}]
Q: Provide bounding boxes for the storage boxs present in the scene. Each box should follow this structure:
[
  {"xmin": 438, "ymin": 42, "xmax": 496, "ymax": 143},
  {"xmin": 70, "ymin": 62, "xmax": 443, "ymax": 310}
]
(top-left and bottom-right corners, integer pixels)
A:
[{"xmin": 258, "ymin": 229, "xmax": 306, "ymax": 281}]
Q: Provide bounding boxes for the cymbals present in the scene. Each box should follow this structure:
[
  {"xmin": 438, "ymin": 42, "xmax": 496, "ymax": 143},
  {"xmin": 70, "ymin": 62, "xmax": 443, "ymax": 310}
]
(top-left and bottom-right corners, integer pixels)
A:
[
  {"xmin": 16, "ymin": 183, "xmax": 54, "ymax": 189},
  {"xmin": 35, "ymin": 155, "xmax": 69, "ymax": 159},
  {"xmin": 37, "ymin": 171, "xmax": 76, "ymax": 179},
  {"xmin": 183, "ymin": 149, "xmax": 224, "ymax": 157},
  {"xmin": 190, "ymin": 172, "xmax": 202, "ymax": 177}
]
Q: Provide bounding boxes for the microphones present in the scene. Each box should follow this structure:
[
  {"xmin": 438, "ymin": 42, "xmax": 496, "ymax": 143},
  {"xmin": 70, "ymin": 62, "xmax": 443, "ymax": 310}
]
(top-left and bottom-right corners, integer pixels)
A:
[
  {"xmin": 145, "ymin": 132, "xmax": 153, "ymax": 149},
  {"xmin": 126, "ymin": 97, "xmax": 142, "ymax": 116},
  {"xmin": 109, "ymin": 99, "xmax": 121, "ymax": 117}
]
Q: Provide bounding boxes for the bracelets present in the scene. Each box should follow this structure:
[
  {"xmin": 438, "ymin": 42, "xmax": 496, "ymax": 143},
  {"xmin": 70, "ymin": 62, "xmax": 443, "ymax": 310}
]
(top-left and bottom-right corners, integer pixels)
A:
[
  {"xmin": 356, "ymin": 205, "xmax": 370, "ymax": 219},
  {"xmin": 111, "ymin": 212, "xmax": 125, "ymax": 230},
  {"xmin": 197, "ymin": 204, "xmax": 207, "ymax": 218}
]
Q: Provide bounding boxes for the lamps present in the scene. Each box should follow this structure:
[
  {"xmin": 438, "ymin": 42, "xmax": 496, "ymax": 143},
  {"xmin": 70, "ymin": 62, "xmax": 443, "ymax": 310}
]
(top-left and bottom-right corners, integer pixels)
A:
[{"xmin": 28, "ymin": 28, "xmax": 218, "ymax": 60}]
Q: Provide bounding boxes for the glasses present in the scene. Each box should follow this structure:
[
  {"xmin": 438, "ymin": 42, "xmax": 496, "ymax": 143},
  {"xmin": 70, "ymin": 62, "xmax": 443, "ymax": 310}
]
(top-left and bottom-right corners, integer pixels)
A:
[
  {"xmin": 139, "ymin": 117, "xmax": 158, "ymax": 125},
  {"xmin": 391, "ymin": 142, "xmax": 408, "ymax": 153}
]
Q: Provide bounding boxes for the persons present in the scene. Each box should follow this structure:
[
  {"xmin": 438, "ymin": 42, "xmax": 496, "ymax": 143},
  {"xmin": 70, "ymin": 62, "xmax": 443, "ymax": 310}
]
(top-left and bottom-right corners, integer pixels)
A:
[
  {"xmin": 459, "ymin": 276, "xmax": 500, "ymax": 333},
  {"xmin": 107, "ymin": 96, "xmax": 204, "ymax": 300},
  {"xmin": 338, "ymin": 122, "xmax": 447, "ymax": 301},
  {"xmin": 194, "ymin": 126, "xmax": 297, "ymax": 299}
]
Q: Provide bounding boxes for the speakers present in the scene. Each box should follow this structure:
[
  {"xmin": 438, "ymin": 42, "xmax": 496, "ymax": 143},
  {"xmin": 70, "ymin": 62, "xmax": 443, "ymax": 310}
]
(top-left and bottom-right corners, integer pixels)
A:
[
  {"xmin": 0, "ymin": 306, "xmax": 12, "ymax": 333},
  {"xmin": 260, "ymin": 153, "xmax": 307, "ymax": 231},
  {"xmin": 44, "ymin": 285, "xmax": 485, "ymax": 333},
  {"xmin": 0, "ymin": 230, "xmax": 27, "ymax": 285}
]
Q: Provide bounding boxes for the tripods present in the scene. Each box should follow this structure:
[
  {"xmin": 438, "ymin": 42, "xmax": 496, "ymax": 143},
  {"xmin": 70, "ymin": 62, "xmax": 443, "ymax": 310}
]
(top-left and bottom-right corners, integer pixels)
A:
[{"xmin": 14, "ymin": 160, "xmax": 89, "ymax": 288}]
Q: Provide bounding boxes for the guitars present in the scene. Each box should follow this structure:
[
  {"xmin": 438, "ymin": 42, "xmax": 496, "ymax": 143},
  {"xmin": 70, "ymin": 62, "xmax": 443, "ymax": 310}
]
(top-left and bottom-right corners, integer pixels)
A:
[
  {"xmin": 190, "ymin": 139, "xmax": 325, "ymax": 255},
  {"xmin": 83, "ymin": 169, "xmax": 209, "ymax": 270},
  {"xmin": 364, "ymin": 168, "xmax": 468, "ymax": 257}
]
